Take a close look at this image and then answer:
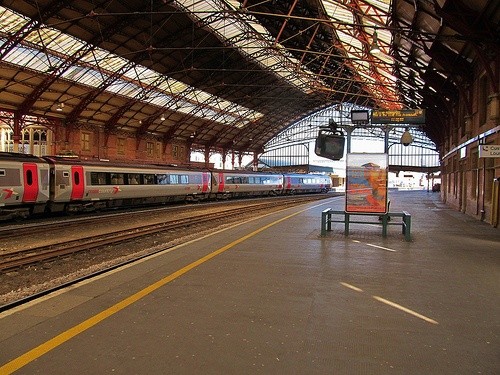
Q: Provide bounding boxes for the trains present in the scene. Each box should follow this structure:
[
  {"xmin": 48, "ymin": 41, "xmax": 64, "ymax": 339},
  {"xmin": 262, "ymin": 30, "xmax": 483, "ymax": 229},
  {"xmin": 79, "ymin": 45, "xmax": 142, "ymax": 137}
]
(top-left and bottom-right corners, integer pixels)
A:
[{"xmin": 0, "ymin": 151, "xmax": 333, "ymax": 219}]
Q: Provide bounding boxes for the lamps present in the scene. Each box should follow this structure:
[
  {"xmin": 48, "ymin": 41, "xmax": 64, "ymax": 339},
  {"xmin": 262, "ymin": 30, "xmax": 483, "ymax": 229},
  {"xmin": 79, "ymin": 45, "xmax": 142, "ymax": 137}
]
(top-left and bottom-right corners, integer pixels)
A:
[
  {"xmin": 249, "ymin": 135, "xmax": 253, "ymax": 141},
  {"xmin": 369, "ymin": 28, "xmax": 382, "ymax": 55},
  {"xmin": 56, "ymin": 103, "xmax": 63, "ymax": 111},
  {"xmin": 190, "ymin": 132, "xmax": 195, "ymax": 138},
  {"xmin": 160, "ymin": 113, "xmax": 166, "ymax": 120}
]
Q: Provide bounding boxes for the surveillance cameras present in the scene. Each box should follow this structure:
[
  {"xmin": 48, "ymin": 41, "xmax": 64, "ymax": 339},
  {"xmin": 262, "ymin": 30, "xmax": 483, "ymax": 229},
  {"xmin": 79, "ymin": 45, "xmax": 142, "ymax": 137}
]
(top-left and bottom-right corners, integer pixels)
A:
[{"xmin": 400, "ymin": 132, "xmax": 412, "ymax": 146}]
